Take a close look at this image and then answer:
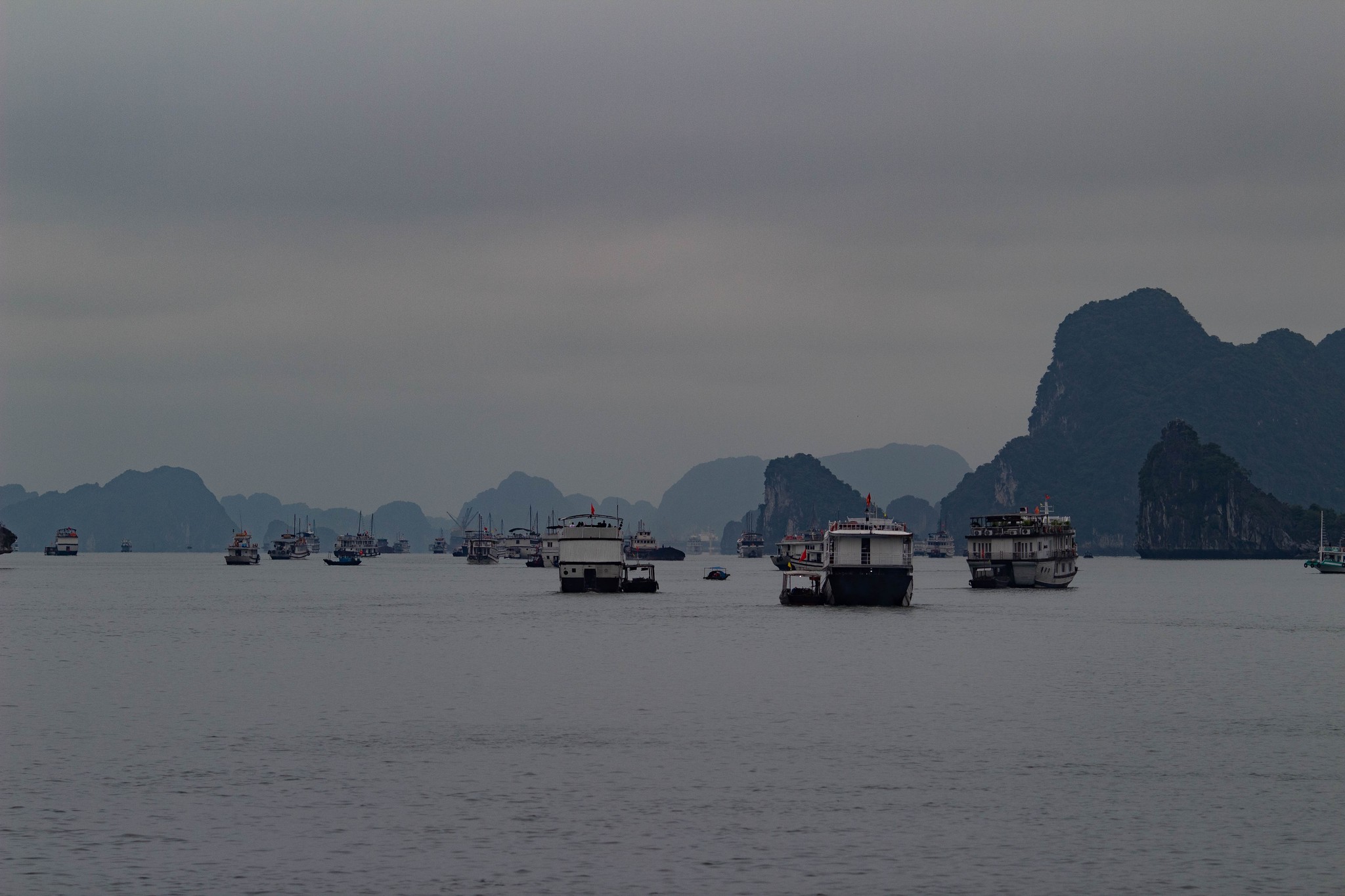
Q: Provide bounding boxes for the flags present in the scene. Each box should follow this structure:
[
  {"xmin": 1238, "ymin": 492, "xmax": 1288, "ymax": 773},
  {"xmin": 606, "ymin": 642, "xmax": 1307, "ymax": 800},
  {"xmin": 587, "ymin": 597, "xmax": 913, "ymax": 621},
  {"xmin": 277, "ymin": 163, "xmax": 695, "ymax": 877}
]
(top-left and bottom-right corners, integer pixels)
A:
[
  {"xmin": 1040, "ymin": 503, "xmax": 1042, "ymax": 506},
  {"xmin": 591, "ymin": 503, "xmax": 595, "ymax": 515},
  {"xmin": 495, "ymin": 529, "xmax": 497, "ymax": 531},
  {"xmin": 1045, "ymin": 494, "xmax": 1050, "ymax": 499},
  {"xmin": 244, "ymin": 530, "xmax": 247, "ymax": 535},
  {"xmin": 637, "ymin": 545, "xmax": 639, "ymax": 551},
  {"xmin": 366, "ymin": 531, "xmax": 368, "ymax": 534},
  {"xmin": 643, "ymin": 522, "xmax": 645, "ymax": 526},
  {"xmin": 360, "ymin": 551, "xmax": 363, "ymax": 555},
  {"xmin": 492, "ymin": 531, "xmax": 493, "ymax": 532},
  {"xmin": 1035, "ymin": 506, "xmax": 1039, "ymax": 514},
  {"xmin": 485, "ymin": 527, "xmax": 487, "ymax": 530},
  {"xmin": 799, "ymin": 549, "xmax": 806, "ymax": 562},
  {"xmin": 866, "ymin": 493, "xmax": 871, "ymax": 506}
]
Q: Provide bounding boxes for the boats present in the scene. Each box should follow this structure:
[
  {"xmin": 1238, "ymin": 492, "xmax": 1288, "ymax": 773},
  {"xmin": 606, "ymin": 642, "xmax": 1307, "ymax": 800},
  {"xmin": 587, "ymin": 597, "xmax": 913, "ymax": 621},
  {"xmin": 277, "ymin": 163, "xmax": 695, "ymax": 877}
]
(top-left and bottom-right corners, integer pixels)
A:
[
  {"xmin": 1304, "ymin": 510, "xmax": 1345, "ymax": 574},
  {"xmin": 925, "ymin": 510, "xmax": 955, "ymax": 558},
  {"xmin": 703, "ymin": 566, "xmax": 731, "ymax": 581},
  {"xmin": 120, "ymin": 536, "xmax": 133, "ymax": 553},
  {"xmin": 965, "ymin": 498, "xmax": 1079, "ymax": 590},
  {"xmin": 44, "ymin": 527, "xmax": 79, "ymax": 556},
  {"xmin": 820, "ymin": 496, "xmax": 914, "ymax": 608},
  {"xmin": 1083, "ymin": 550, "xmax": 1094, "ymax": 558},
  {"xmin": 770, "ymin": 499, "xmax": 834, "ymax": 572},
  {"xmin": 224, "ymin": 509, "xmax": 260, "ymax": 566},
  {"xmin": 912, "ymin": 537, "xmax": 928, "ymax": 556},
  {"xmin": 736, "ymin": 511, "xmax": 765, "ymax": 558},
  {"xmin": 778, "ymin": 570, "xmax": 828, "ymax": 607},
  {"xmin": 266, "ymin": 495, "xmax": 686, "ymax": 595}
]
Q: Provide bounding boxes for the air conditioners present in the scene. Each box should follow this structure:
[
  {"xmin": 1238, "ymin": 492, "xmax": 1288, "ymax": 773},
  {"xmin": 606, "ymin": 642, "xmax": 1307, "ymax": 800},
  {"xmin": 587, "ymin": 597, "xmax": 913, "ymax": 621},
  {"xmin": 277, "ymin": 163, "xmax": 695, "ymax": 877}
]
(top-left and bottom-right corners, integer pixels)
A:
[
  {"xmin": 974, "ymin": 530, "xmax": 982, "ymax": 536},
  {"xmin": 1022, "ymin": 529, "xmax": 1030, "ymax": 534},
  {"xmin": 984, "ymin": 530, "xmax": 992, "ymax": 536},
  {"xmin": 1010, "ymin": 531, "xmax": 1017, "ymax": 535}
]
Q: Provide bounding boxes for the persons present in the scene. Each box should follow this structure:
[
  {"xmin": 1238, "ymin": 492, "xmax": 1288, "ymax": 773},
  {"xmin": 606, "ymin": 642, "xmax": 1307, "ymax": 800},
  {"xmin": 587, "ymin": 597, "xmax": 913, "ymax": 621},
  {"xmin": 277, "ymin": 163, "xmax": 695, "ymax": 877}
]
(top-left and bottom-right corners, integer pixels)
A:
[
  {"xmin": 992, "ymin": 523, "xmax": 996, "ymax": 527},
  {"xmin": 507, "ymin": 533, "xmax": 522, "ymax": 539},
  {"xmin": 713, "ymin": 571, "xmax": 721, "ymax": 578},
  {"xmin": 597, "ymin": 520, "xmax": 606, "ymax": 527},
  {"xmin": 607, "ymin": 523, "xmax": 611, "ymax": 528},
  {"xmin": 1329, "ymin": 542, "xmax": 1332, "ymax": 548},
  {"xmin": 1341, "ymin": 544, "xmax": 1344, "ymax": 547},
  {"xmin": 569, "ymin": 522, "xmax": 584, "ymax": 527},
  {"xmin": 274, "ymin": 543, "xmax": 285, "ymax": 550},
  {"xmin": 1073, "ymin": 543, "xmax": 1076, "ymax": 548},
  {"xmin": 662, "ymin": 544, "xmax": 664, "ymax": 548}
]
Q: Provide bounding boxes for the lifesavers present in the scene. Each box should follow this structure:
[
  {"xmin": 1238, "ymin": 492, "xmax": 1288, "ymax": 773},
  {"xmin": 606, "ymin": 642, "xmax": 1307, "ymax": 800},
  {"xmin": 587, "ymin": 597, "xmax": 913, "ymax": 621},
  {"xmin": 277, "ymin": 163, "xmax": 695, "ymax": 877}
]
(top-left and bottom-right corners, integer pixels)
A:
[
  {"xmin": 308, "ymin": 553, "xmax": 310, "ymax": 556},
  {"xmin": 365, "ymin": 553, "xmax": 366, "ymax": 556},
  {"xmin": 272, "ymin": 550, "xmax": 275, "ymax": 554},
  {"xmin": 473, "ymin": 556, "xmax": 477, "ymax": 560},
  {"xmin": 287, "ymin": 551, "xmax": 290, "ymax": 554},
  {"xmin": 258, "ymin": 553, "xmax": 259, "ymax": 558},
  {"xmin": 479, "ymin": 556, "xmax": 483, "ymax": 560},
  {"xmin": 282, "ymin": 551, "xmax": 285, "ymax": 554},
  {"xmin": 278, "ymin": 552, "xmax": 281, "ymax": 555},
  {"xmin": 379, "ymin": 553, "xmax": 380, "ymax": 555},
  {"xmin": 746, "ymin": 536, "xmax": 748, "ymax": 538},
  {"xmin": 512, "ymin": 554, "xmax": 515, "ymax": 557},
  {"xmin": 256, "ymin": 559, "xmax": 258, "ymax": 563},
  {"xmin": 483, "ymin": 555, "xmax": 487, "ymax": 559},
  {"xmin": 371, "ymin": 553, "xmax": 372, "ymax": 555},
  {"xmin": 267, "ymin": 550, "xmax": 271, "ymax": 554},
  {"xmin": 376, "ymin": 552, "xmax": 377, "ymax": 555},
  {"xmin": 469, "ymin": 556, "xmax": 473, "ymax": 560}
]
